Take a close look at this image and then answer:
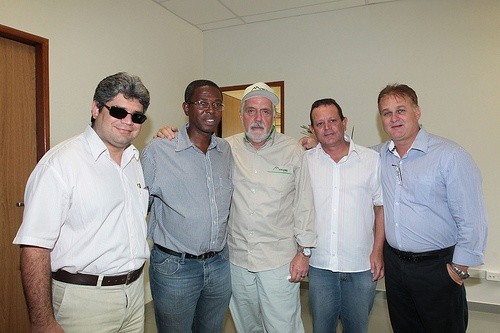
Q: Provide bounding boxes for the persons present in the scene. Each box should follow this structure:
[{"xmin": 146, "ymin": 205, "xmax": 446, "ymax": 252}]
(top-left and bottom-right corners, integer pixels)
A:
[
  {"xmin": 304, "ymin": 98, "xmax": 385, "ymax": 333},
  {"xmin": 140, "ymin": 79, "xmax": 234, "ymax": 333},
  {"xmin": 153, "ymin": 82, "xmax": 318, "ymax": 333},
  {"xmin": 12, "ymin": 73, "xmax": 151, "ymax": 333},
  {"xmin": 299, "ymin": 84, "xmax": 490, "ymax": 333}
]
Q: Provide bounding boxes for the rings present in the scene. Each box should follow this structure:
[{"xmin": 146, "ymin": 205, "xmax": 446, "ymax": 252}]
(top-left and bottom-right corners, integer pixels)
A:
[{"xmin": 301, "ymin": 275, "xmax": 305, "ymax": 278}]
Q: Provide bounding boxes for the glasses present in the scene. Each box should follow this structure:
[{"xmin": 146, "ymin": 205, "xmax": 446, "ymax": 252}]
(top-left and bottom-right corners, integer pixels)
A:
[
  {"xmin": 187, "ymin": 100, "xmax": 226, "ymax": 112},
  {"xmin": 100, "ymin": 102, "xmax": 148, "ymax": 125}
]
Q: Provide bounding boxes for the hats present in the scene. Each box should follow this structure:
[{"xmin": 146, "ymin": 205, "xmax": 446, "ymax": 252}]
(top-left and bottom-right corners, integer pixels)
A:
[{"xmin": 241, "ymin": 82, "xmax": 280, "ymax": 106}]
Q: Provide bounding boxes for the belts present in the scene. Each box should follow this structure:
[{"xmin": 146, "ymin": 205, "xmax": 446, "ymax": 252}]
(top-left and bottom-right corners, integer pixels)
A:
[
  {"xmin": 385, "ymin": 238, "xmax": 454, "ymax": 263},
  {"xmin": 51, "ymin": 260, "xmax": 147, "ymax": 286},
  {"xmin": 154, "ymin": 243, "xmax": 215, "ymax": 259}
]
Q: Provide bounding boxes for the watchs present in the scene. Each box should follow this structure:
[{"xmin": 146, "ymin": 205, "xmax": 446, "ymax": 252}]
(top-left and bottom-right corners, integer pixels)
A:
[
  {"xmin": 299, "ymin": 246, "xmax": 312, "ymax": 257},
  {"xmin": 450, "ymin": 262, "xmax": 470, "ymax": 279}
]
